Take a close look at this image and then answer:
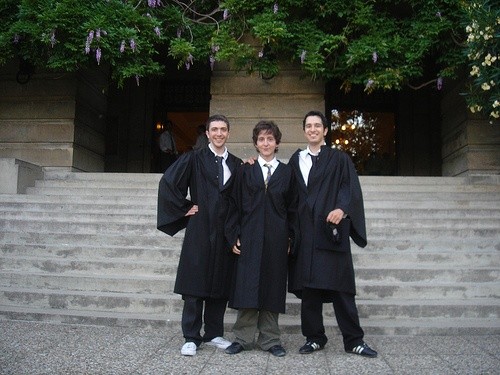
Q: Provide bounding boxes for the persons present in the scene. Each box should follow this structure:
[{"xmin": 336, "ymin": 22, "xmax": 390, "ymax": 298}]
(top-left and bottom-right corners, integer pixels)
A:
[
  {"xmin": 156, "ymin": 114, "xmax": 245, "ymax": 355},
  {"xmin": 346, "ymin": 148, "xmax": 396, "ymax": 176},
  {"xmin": 245, "ymin": 111, "xmax": 378, "ymax": 358},
  {"xmin": 194, "ymin": 124, "xmax": 210, "ymax": 152},
  {"xmin": 159, "ymin": 120, "xmax": 177, "ymax": 173},
  {"xmin": 223, "ymin": 119, "xmax": 301, "ymax": 358}
]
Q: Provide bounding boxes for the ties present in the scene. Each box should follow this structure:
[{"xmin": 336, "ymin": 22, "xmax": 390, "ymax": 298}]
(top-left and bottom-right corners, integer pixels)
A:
[
  {"xmin": 307, "ymin": 154, "xmax": 318, "ymax": 189},
  {"xmin": 215, "ymin": 155, "xmax": 225, "ymax": 187},
  {"xmin": 263, "ymin": 164, "xmax": 271, "ymax": 187}
]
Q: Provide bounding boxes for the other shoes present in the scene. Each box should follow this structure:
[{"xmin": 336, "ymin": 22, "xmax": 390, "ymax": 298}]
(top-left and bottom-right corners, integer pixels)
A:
[
  {"xmin": 225, "ymin": 342, "xmax": 243, "ymax": 354},
  {"xmin": 181, "ymin": 341, "xmax": 197, "ymax": 356},
  {"xmin": 205, "ymin": 336, "xmax": 231, "ymax": 348},
  {"xmin": 269, "ymin": 345, "xmax": 286, "ymax": 357}
]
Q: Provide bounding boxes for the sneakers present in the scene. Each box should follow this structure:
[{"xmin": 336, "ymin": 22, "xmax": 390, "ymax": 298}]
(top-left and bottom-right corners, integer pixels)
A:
[
  {"xmin": 345, "ymin": 342, "xmax": 378, "ymax": 358},
  {"xmin": 298, "ymin": 340, "xmax": 324, "ymax": 354}
]
